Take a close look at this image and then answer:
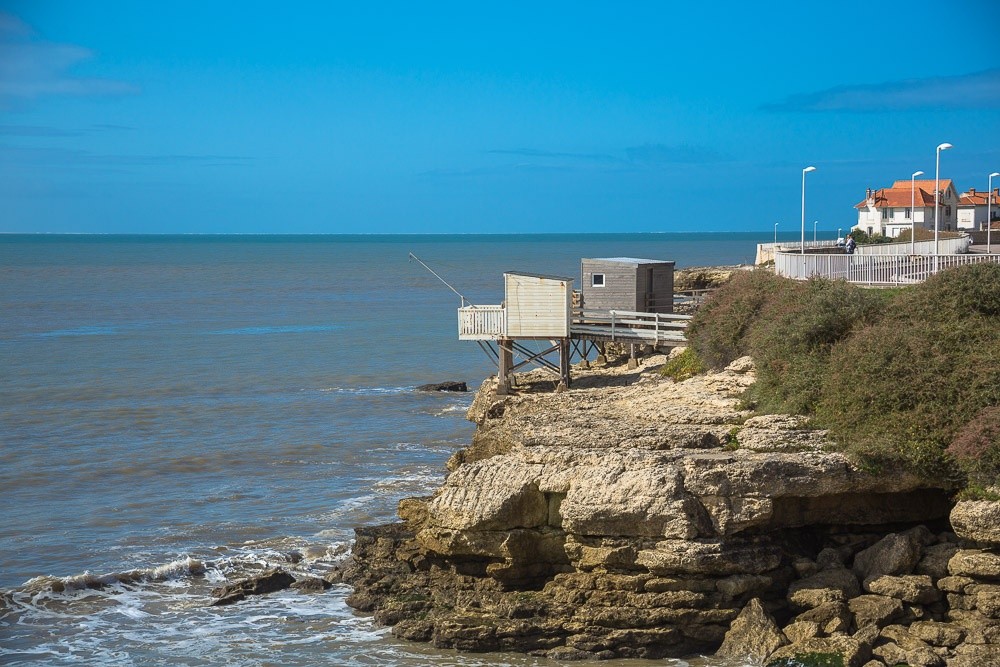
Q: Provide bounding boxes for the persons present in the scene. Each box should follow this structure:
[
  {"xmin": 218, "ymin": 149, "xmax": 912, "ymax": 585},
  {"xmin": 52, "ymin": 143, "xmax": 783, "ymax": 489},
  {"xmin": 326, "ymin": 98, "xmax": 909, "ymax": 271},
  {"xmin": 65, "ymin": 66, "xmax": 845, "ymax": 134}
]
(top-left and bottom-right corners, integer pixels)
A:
[{"xmin": 846, "ymin": 234, "xmax": 856, "ymax": 254}]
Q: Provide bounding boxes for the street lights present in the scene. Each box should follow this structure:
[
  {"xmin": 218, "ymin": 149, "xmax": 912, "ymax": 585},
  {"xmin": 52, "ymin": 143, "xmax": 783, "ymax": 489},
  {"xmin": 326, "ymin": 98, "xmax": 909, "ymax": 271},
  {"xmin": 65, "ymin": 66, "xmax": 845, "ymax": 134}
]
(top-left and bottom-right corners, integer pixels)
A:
[
  {"xmin": 837, "ymin": 228, "xmax": 842, "ymax": 246},
  {"xmin": 911, "ymin": 170, "xmax": 924, "ymax": 262},
  {"xmin": 774, "ymin": 223, "xmax": 781, "ymax": 243},
  {"xmin": 987, "ymin": 171, "xmax": 999, "ymax": 253},
  {"xmin": 800, "ymin": 166, "xmax": 816, "ymax": 254},
  {"xmin": 933, "ymin": 142, "xmax": 953, "ymax": 272},
  {"xmin": 813, "ymin": 221, "xmax": 818, "ymax": 248}
]
